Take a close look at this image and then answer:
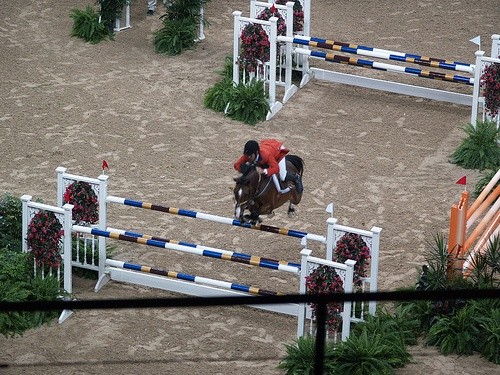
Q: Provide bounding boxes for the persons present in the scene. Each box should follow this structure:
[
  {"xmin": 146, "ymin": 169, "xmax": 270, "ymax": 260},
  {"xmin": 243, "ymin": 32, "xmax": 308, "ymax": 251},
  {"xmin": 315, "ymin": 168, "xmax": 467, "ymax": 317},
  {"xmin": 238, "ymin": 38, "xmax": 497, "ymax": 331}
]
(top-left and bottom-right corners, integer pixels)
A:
[{"xmin": 234, "ymin": 137, "xmax": 306, "ymax": 195}]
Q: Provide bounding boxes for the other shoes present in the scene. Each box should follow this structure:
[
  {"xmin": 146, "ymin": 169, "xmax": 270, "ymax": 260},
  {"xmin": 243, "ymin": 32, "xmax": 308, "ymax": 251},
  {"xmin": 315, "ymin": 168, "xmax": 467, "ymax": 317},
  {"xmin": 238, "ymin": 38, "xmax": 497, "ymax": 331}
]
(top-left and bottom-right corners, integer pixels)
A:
[{"xmin": 146, "ymin": 10, "xmax": 153, "ymax": 15}]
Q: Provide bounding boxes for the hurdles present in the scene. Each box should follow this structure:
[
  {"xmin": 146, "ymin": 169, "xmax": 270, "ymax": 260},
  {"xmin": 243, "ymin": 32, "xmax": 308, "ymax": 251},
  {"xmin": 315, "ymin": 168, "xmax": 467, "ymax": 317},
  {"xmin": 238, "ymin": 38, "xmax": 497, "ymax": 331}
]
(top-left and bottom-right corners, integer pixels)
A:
[
  {"xmin": 233, "ymin": 0, "xmax": 500, "ymax": 132},
  {"xmin": 20, "ymin": 166, "xmax": 383, "ymax": 350}
]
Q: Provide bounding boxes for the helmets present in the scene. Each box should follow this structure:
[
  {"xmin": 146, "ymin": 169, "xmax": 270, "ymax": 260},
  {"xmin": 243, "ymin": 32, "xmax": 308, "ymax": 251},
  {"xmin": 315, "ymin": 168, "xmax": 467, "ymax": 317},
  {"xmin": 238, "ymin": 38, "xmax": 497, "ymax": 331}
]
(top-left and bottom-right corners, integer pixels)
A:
[{"xmin": 244, "ymin": 140, "xmax": 259, "ymax": 156}]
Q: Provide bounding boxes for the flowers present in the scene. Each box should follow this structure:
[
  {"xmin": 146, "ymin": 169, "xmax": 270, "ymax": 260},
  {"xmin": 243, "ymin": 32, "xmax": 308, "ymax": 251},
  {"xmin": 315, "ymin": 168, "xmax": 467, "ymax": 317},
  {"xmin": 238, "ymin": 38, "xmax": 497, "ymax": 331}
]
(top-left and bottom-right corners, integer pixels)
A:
[
  {"xmin": 276, "ymin": 0, "xmax": 305, "ymax": 32},
  {"xmin": 334, "ymin": 232, "xmax": 371, "ymax": 288},
  {"xmin": 305, "ymin": 265, "xmax": 344, "ymax": 332},
  {"xmin": 480, "ymin": 64, "xmax": 500, "ymax": 118},
  {"xmin": 63, "ymin": 182, "xmax": 99, "ymax": 225},
  {"xmin": 24, "ymin": 210, "xmax": 64, "ymax": 266},
  {"xmin": 256, "ymin": 6, "xmax": 286, "ymax": 32},
  {"xmin": 236, "ymin": 22, "xmax": 270, "ymax": 71}
]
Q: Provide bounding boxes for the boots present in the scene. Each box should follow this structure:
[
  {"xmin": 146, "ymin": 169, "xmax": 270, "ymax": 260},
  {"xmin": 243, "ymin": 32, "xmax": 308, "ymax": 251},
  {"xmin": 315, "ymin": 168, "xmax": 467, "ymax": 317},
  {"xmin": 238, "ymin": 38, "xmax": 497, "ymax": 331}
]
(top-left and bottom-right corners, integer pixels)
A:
[{"xmin": 281, "ymin": 171, "xmax": 303, "ymax": 193}]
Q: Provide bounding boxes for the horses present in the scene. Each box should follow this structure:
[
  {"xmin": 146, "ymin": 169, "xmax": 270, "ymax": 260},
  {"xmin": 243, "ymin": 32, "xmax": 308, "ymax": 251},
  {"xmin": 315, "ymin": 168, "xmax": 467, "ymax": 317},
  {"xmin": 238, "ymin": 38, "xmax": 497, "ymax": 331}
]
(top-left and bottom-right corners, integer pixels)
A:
[{"xmin": 231, "ymin": 155, "xmax": 305, "ymax": 224}]
